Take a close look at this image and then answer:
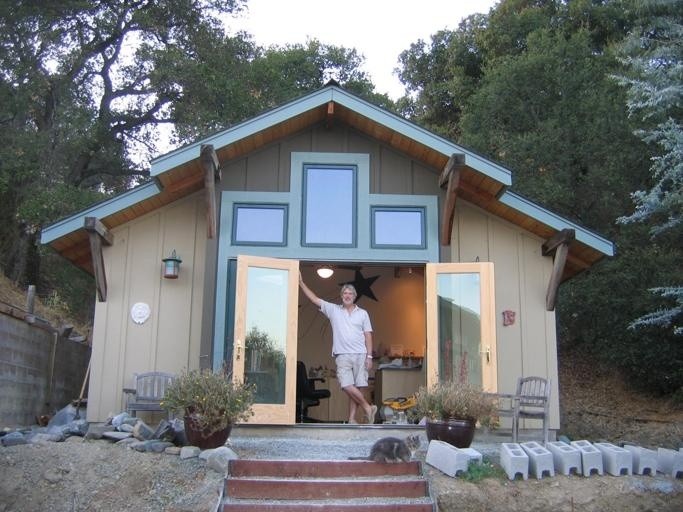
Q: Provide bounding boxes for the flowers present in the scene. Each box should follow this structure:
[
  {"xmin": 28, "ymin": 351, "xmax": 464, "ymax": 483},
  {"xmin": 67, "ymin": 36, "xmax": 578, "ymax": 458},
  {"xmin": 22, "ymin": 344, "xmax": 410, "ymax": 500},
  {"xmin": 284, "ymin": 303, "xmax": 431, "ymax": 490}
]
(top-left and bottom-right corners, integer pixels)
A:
[{"xmin": 160, "ymin": 360, "xmax": 258, "ymax": 433}]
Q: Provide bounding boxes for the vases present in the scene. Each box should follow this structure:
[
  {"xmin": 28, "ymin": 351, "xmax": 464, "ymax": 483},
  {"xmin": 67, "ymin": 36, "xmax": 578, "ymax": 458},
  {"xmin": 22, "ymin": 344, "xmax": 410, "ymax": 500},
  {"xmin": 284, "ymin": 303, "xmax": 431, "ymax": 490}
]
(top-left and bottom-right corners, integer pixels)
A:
[{"xmin": 183, "ymin": 406, "xmax": 232, "ymax": 448}]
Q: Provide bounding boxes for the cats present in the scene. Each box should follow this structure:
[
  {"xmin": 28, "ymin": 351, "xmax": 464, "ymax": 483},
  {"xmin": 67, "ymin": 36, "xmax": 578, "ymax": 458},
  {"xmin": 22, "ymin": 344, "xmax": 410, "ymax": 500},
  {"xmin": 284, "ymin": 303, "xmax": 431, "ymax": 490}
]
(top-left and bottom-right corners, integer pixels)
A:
[{"xmin": 347, "ymin": 435, "xmax": 421, "ymax": 463}]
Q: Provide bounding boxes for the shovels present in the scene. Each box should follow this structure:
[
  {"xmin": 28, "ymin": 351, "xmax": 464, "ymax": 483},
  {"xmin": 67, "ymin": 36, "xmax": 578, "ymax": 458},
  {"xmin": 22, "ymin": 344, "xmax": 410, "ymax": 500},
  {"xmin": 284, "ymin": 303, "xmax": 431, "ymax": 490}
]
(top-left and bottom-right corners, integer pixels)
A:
[{"xmin": 72, "ymin": 360, "xmax": 91, "ymax": 420}]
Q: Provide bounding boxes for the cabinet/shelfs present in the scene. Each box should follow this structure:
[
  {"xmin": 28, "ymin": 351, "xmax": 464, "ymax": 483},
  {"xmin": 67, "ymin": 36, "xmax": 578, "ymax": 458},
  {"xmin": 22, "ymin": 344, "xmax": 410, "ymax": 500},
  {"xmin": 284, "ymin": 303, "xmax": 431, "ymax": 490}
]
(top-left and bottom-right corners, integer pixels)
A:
[{"xmin": 308, "ymin": 370, "xmax": 426, "ymax": 424}]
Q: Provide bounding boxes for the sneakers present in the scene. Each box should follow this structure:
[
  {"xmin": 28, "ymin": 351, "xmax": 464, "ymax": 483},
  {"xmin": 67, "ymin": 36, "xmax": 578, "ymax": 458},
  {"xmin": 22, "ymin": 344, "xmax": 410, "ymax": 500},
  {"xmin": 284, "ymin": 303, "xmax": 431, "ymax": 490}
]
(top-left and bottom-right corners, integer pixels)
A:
[
  {"xmin": 366, "ymin": 405, "xmax": 377, "ymax": 424},
  {"xmin": 348, "ymin": 419, "xmax": 359, "ymax": 424}
]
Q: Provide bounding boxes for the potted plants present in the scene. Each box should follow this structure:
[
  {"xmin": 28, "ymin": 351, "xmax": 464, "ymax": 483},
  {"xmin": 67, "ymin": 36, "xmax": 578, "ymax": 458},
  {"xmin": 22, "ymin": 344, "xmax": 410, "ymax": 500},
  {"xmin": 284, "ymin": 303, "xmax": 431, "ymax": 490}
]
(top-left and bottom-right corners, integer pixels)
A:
[{"xmin": 409, "ymin": 365, "xmax": 504, "ymax": 448}]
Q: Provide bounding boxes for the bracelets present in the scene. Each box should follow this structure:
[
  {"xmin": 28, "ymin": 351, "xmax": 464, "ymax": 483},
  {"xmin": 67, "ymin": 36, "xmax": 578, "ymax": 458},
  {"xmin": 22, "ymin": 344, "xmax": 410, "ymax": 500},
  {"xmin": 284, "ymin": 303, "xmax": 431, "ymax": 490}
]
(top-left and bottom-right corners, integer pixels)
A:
[{"xmin": 366, "ymin": 354, "xmax": 373, "ymax": 359}]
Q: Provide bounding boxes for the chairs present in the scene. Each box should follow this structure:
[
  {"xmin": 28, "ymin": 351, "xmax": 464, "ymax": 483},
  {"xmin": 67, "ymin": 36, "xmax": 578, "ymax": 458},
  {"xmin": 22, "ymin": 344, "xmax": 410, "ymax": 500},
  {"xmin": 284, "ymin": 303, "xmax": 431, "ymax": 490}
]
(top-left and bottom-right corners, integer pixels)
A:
[
  {"xmin": 482, "ymin": 376, "xmax": 551, "ymax": 444},
  {"xmin": 122, "ymin": 371, "xmax": 175, "ymax": 421},
  {"xmin": 296, "ymin": 360, "xmax": 330, "ymax": 423}
]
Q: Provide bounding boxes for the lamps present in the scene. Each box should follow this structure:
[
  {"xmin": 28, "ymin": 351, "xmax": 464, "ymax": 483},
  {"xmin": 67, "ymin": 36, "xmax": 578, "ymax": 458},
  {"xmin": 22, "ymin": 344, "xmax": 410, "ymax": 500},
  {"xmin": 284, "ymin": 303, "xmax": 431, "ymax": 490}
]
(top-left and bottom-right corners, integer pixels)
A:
[
  {"xmin": 317, "ymin": 265, "xmax": 334, "ymax": 279},
  {"xmin": 162, "ymin": 248, "xmax": 182, "ymax": 279}
]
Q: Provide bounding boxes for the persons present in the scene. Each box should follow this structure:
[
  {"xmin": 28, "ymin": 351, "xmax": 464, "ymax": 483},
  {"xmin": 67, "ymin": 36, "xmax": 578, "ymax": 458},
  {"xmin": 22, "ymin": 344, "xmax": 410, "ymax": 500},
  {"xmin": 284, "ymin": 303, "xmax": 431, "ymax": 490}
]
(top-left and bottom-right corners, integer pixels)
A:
[{"xmin": 299, "ymin": 270, "xmax": 377, "ymax": 425}]
